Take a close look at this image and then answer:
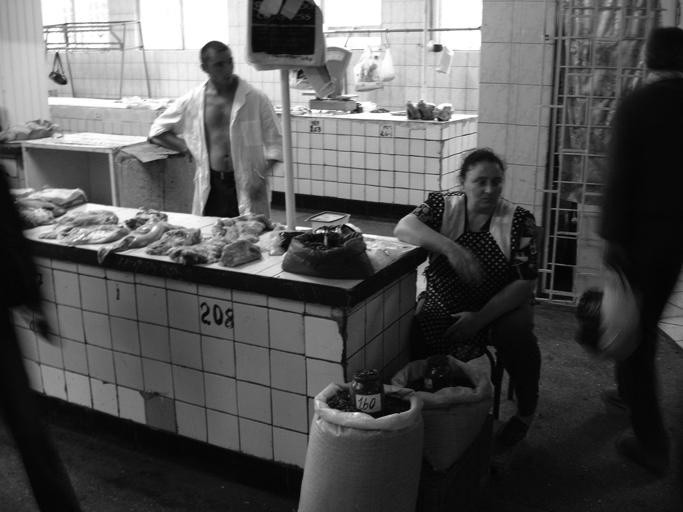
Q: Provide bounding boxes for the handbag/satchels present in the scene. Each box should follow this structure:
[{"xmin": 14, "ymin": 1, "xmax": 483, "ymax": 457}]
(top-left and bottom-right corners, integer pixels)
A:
[{"xmin": 49, "ymin": 54, "xmax": 67, "ymax": 84}]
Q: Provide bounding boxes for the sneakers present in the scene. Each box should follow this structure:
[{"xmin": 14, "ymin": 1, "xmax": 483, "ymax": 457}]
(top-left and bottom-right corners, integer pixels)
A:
[
  {"xmin": 615, "ymin": 438, "xmax": 669, "ymax": 474},
  {"xmin": 599, "ymin": 389, "xmax": 626, "ymax": 410}
]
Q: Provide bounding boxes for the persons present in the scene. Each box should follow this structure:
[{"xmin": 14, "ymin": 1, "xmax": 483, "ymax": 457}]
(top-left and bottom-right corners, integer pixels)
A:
[
  {"xmin": 391, "ymin": 148, "xmax": 544, "ymax": 468},
  {"xmin": 589, "ymin": 24, "xmax": 682, "ymax": 481},
  {"xmin": 1, "ymin": 109, "xmax": 76, "ymax": 509},
  {"xmin": 147, "ymin": 39, "xmax": 282, "ymax": 225}
]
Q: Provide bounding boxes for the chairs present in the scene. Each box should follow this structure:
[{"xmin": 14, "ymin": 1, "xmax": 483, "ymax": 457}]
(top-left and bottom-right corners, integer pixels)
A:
[{"xmin": 409, "ymin": 223, "xmax": 543, "ymax": 446}]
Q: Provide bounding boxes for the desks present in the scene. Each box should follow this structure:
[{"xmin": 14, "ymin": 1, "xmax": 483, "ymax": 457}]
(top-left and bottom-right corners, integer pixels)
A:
[{"xmin": 21, "ymin": 128, "xmax": 164, "ymax": 212}]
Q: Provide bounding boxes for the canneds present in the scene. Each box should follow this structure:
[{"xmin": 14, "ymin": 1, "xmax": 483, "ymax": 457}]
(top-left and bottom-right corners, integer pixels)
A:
[
  {"xmin": 424, "ymin": 356, "xmax": 449, "ymax": 393},
  {"xmin": 353, "ymin": 369, "xmax": 386, "ymax": 417}
]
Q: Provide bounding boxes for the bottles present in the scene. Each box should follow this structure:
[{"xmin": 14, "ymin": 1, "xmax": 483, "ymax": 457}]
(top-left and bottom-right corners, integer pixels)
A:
[
  {"xmin": 421, "ymin": 356, "xmax": 453, "ymax": 391},
  {"xmin": 350, "ymin": 366, "xmax": 387, "ymax": 418}
]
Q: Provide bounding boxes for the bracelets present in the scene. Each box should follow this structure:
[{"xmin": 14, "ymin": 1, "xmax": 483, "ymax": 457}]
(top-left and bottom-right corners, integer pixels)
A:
[{"xmin": 254, "ymin": 170, "xmax": 265, "ymax": 182}]
